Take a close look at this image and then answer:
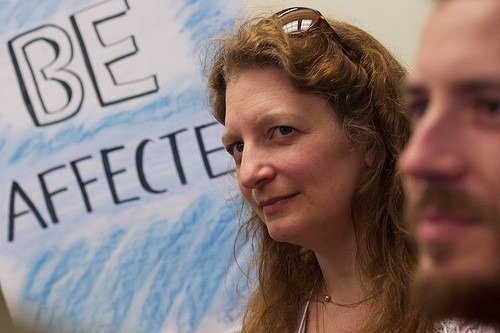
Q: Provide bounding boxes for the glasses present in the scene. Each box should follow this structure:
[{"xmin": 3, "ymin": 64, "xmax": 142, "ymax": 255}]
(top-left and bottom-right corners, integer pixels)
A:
[{"xmin": 269, "ymin": 6, "xmax": 360, "ymax": 62}]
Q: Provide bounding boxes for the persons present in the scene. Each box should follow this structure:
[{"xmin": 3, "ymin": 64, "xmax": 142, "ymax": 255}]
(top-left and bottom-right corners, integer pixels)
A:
[
  {"xmin": 391, "ymin": 0, "xmax": 500, "ymax": 333},
  {"xmin": 198, "ymin": 6, "xmax": 417, "ymax": 333}
]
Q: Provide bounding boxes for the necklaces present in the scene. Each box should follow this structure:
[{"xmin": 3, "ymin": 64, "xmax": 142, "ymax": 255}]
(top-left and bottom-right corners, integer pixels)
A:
[{"xmin": 322, "ymin": 279, "xmax": 384, "ymax": 306}]
